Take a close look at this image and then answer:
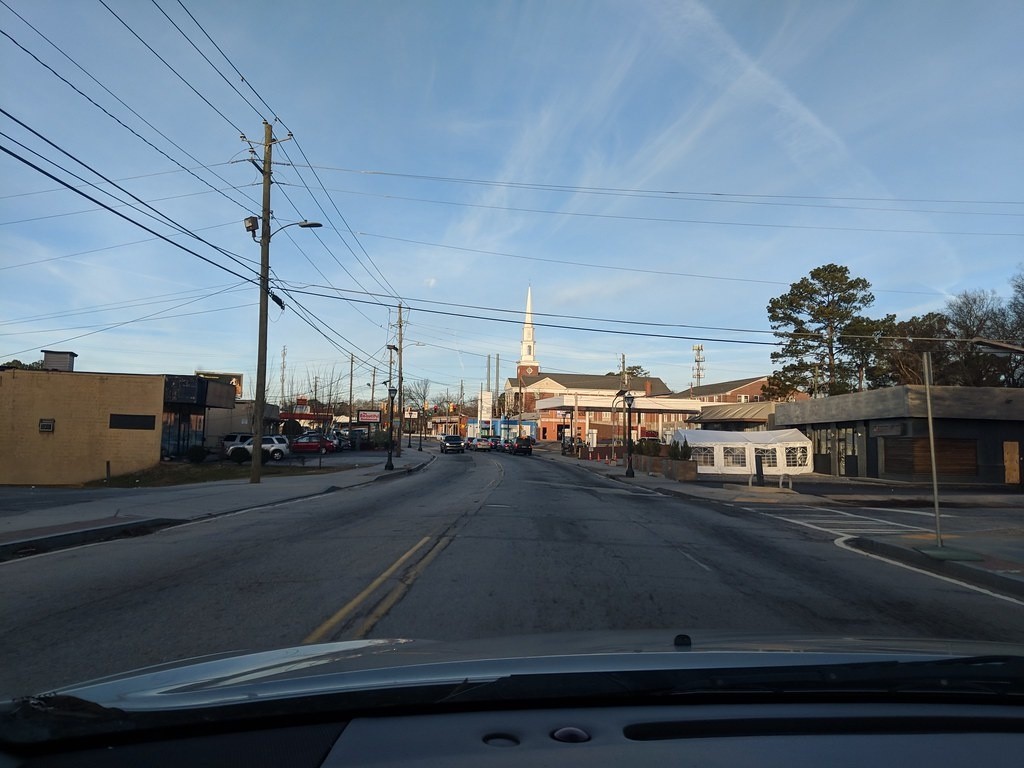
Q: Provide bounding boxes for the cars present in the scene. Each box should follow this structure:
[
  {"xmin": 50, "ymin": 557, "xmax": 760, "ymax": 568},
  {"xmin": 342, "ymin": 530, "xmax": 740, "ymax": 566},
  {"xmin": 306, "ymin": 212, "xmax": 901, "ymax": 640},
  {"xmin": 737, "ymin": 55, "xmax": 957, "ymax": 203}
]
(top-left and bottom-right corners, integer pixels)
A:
[
  {"xmin": 339, "ymin": 429, "xmax": 350, "ymax": 436},
  {"xmin": 324, "ymin": 436, "xmax": 350, "ymax": 453},
  {"xmin": 470, "ymin": 438, "xmax": 491, "ymax": 452},
  {"xmin": 488, "ymin": 438, "xmax": 500, "ymax": 449},
  {"xmin": 464, "ymin": 436, "xmax": 476, "ymax": 449},
  {"xmin": 289, "ymin": 434, "xmax": 334, "ymax": 454},
  {"xmin": 499, "ymin": 439, "xmax": 513, "ymax": 452}
]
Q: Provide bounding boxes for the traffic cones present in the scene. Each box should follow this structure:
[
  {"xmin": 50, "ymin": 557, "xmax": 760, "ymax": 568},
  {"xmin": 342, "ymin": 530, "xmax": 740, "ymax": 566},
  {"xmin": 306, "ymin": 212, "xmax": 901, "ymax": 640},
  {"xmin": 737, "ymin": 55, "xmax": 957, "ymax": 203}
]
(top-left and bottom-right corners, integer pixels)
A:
[
  {"xmin": 596, "ymin": 453, "xmax": 601, "ymax": 462},
  {"xmin": 605, "ymin": 454, "xmax": 610, "ymax": 464},
  {"xmin": 383, "ymin": 386, "xmax": 397, "ymax": 471},
  {"xmin": 614, "ymin": 453, "xmax": 618, "ymax": 461}
]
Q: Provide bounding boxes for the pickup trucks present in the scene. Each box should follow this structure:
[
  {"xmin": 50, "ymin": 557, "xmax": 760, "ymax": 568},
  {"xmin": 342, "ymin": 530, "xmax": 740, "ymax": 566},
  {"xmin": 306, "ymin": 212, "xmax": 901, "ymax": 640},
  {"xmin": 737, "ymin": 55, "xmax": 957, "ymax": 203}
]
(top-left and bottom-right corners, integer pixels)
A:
[{"xmin": 440, "ymin": 435, "xmax": 465, "ymax": 454}]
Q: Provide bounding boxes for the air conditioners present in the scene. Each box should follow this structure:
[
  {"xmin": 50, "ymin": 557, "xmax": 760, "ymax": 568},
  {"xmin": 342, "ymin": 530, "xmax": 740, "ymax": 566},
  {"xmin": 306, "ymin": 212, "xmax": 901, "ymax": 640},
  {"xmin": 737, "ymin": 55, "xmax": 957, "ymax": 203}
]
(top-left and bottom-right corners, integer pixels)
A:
[{"xmin": 788, "ymin": 395, "xmax": 795, "ymax": 403}]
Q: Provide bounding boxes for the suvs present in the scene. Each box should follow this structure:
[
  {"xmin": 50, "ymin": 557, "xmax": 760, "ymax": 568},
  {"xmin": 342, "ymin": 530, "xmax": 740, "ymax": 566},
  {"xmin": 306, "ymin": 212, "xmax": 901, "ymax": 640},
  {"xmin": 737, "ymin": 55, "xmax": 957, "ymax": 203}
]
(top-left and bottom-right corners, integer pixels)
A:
[
  {"xmin": 223, "ymin": 432, "xmax": 254, "ymax": 455},
  {"xmin": 226, "ymin": 434, "xmax": 291, "ymax": 462},
  {"xmin": 509, "ymin": 437, "xmax": 532, "ymax": 457}
]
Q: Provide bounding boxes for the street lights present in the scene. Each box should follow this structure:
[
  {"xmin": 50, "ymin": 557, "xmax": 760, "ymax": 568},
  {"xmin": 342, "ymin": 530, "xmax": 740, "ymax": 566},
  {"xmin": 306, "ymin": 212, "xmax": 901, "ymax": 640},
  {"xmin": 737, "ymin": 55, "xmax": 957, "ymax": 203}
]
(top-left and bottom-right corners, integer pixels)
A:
[{"xmin": 614, "ymin": 387, "xmax": 637, "ymax": 478}]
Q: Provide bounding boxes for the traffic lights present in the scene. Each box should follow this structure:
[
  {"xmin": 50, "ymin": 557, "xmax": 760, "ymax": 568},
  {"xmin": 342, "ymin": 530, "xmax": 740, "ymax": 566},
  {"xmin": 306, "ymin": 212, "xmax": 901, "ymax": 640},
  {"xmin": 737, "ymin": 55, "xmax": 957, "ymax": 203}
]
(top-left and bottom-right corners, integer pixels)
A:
[
  {"xmin": 428, "ymin": 412, "xmax": 432, "ymax": 417},
  {"xmin": 452, "ymin": 404, "xmax": 458, "ymax": 411},
  {"xmin": 433, "ymin": 404, "xmax": 438, "ymax": 414}
]
